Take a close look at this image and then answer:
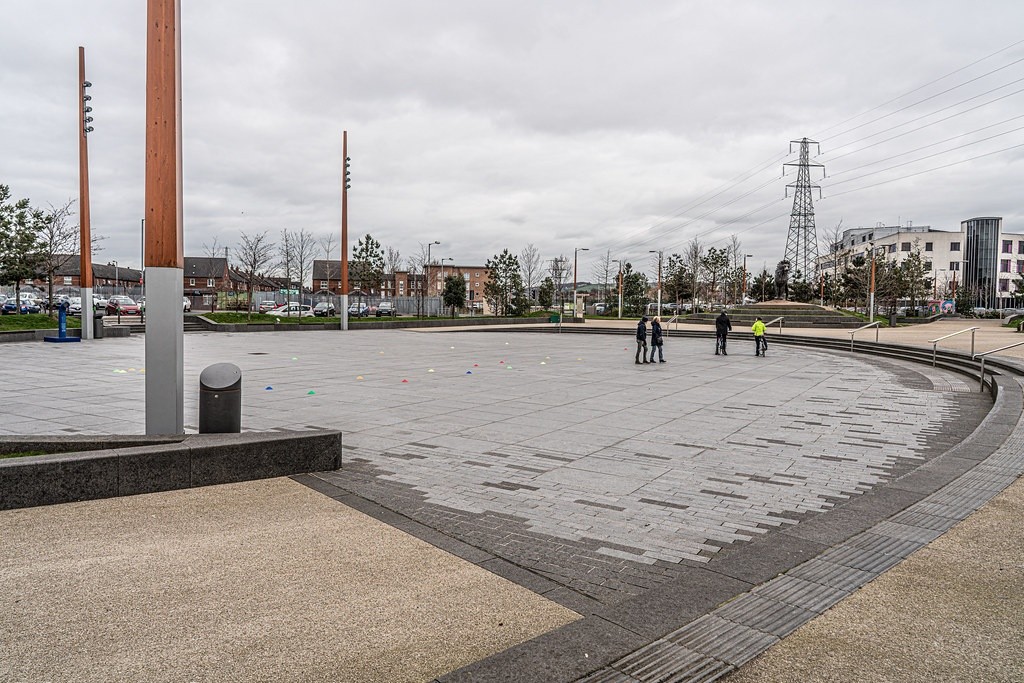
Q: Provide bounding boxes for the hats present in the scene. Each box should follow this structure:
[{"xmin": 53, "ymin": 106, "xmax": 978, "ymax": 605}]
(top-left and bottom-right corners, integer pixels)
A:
[
  {"xmin": 642, "ymin": 317, "xmax": 648, "ymax": 322},
  {"xmin": 721, "ymin": 309, "xmax": 728, "ymax": 313}
]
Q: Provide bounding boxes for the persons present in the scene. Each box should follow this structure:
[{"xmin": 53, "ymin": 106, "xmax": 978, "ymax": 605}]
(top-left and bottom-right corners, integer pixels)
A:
[
  {"xmin": 635, "ymin": 317, "xmax": 649, "ymax": 364},
  {"xmin": 650, "ymin": 316, "xmax": 666, "ymax": 363},
  {"xmin": 752, "ymin": 317, "xmax": 767, "ymax": 356},
  {"xmin": 715, "ymin": 311, "xmax": 732, "ymax": 355}
]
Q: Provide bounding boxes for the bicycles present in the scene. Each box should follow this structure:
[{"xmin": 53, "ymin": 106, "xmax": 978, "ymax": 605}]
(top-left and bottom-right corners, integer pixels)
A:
[{"xmin": 716, "ymin": 331, "xmax": 767, "ymax": 357}]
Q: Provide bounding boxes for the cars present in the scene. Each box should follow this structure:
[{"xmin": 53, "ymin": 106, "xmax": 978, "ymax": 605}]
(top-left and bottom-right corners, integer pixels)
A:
[
  {"xmin": 312, "ymin": 302, "xmax": 336, "ymax": 317},
  {"xmin": 66, "ymin": 296, "xmax": 81, "ymax": 313},
  {"xmin": 2, "ymin": 298, "xmax": 41, "ymax": 314},
  {"xmin": 183, "ymin": 297, "xmax": 191, "ymax": 312},
  {"xmin": 40, "ymin": 295, "xmax": 72, "ymax": 309},
  {"xmin": 0, "ymin": 295, "xmax": 8, "ymax": 306},
  {"xmin": 258, "ymin": 301, "xmax": 277, "ymax": 313},
  {"xmin": 92, "ymin": 294, "xmax": 146, "ymax": 317},
  {"xmin": 13, "ymin": 292, "xmax": 43, "ymax": 305},
  {"xmin": 375, "ymin": 302, "xmax": 397, "ymax": 317},
  {"xmin": 265, "ymin": 305, "xmax": 315, "ymax": 317},
  {"xmin": 348, "ymin": 302, "xmax": 369, "ymax": 318}
]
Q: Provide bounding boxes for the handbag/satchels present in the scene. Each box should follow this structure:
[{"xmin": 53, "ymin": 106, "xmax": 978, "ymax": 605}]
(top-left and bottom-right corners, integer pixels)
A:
[{"xmin": 656, "ymin": 337, "xmax": 662, "ymax": 344}]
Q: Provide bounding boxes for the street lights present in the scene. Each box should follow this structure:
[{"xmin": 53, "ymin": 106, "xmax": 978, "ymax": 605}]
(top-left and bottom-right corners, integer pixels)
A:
[
  {"xmin": 743, "ymin": 254, "xmax": 753, "ymax": 305},
  {"xmin": 427, "ymin": 241, "xmax": 453, "ymax": 318},
  {"xmin": 107, "ymin": 261, "xmax": 118, "ymax": 295},
  {"xmin": 573, "ymin": 248, "xmax": 621, "ymax": 318},
  {"xmin": 78, "ymin": 81, "xmax": 93, "ymax": 340},
  {"xmin": 649, "ymin": 251, "xmax": 660, "ymax": 321},
  {"xmin": 340, "ymin": 157, "xmax": 351, "ymax": 330}
]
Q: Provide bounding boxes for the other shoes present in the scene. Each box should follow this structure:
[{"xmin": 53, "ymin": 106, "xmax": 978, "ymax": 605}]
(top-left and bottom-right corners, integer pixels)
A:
[
  {"xmin": 755, "ymin": 353, "xmax": 759, "ymax": 356},
  {"xmin": 764, "ymin": 346, "xmax": 767, "ymax": 349},
  {"xmin": 643, "ymin": 360, "xmax": 650, "ymax": 364},
  {"xmin": 722, "ymin": 350, "xmax": 727, "ymax": 355},
  {"xmin": 715, "ymin": 352, "xmax": 720, "ymax": 355},
  {"xmin": 660, "ymin": 360, "xmax": 666, "ymax": 363},
  {"xmin": 636, "ymin": 361, "xmax": 643, "ymax": 364},
  {"xmin": 650, "ymin": 359, "xmax": 656, "ymax": 363}
]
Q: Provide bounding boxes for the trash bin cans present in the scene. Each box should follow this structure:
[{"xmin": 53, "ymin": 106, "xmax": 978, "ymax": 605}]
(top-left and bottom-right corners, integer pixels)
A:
[
  {"xmin": 94, "ymin": 313, "xmax": 104, "ymax": 339},
  {"xmin": 451, "ymin": 305, "xmax": 460, "ymax": 317},
  {"xmin": 199, "ymin": 363, "xmax": 241, "ymax": 433},
  {"xmin": 550, "ymin": 314, "xmax": 560, "ymax": 323},
  {"xmin": 906, "ymin": 310, "xmax": 919, "ymax": 317},
  {"xmin": 889, "ymin": 313, "xmax": 898, "ymax": 327}
]
